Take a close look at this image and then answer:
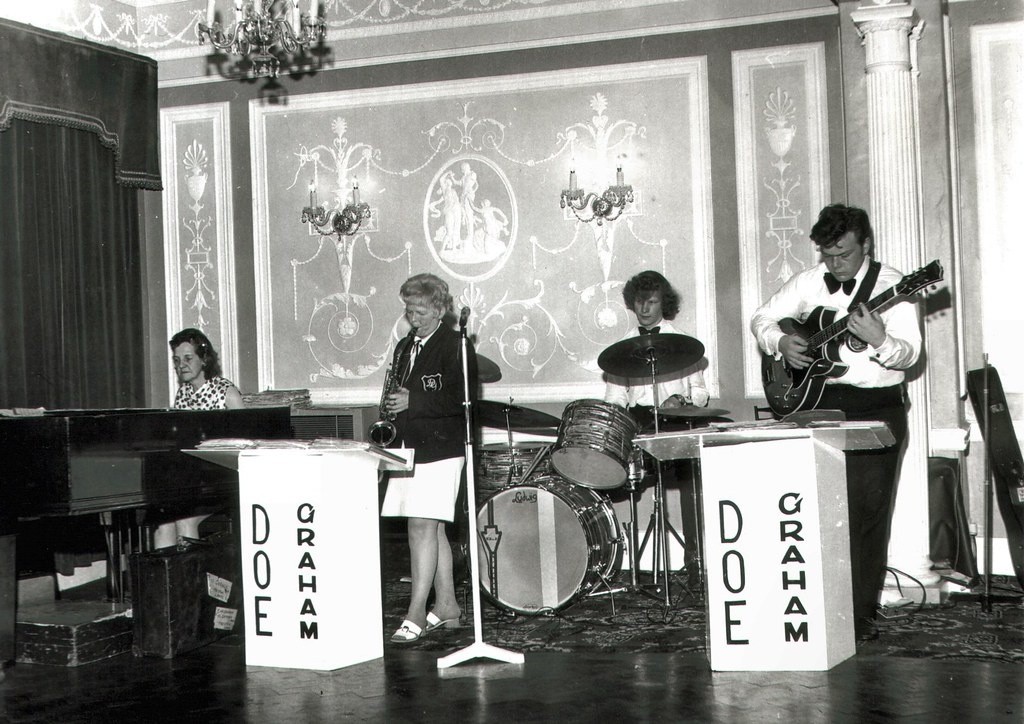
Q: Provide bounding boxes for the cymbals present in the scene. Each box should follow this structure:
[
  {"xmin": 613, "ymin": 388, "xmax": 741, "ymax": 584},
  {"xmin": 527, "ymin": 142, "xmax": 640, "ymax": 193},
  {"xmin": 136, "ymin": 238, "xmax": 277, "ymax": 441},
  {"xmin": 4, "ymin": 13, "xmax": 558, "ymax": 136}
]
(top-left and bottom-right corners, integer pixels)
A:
[
  {"xmin": 649, "ymin": 403, "xmax": 730, "ymax": 420},
  {"xmin": 596, "ymin": 333, "xmax": 706, "ymax": 378},
  {"xmin": 472, "ymin": 397, "xmax": 563, "ymax": 432}
]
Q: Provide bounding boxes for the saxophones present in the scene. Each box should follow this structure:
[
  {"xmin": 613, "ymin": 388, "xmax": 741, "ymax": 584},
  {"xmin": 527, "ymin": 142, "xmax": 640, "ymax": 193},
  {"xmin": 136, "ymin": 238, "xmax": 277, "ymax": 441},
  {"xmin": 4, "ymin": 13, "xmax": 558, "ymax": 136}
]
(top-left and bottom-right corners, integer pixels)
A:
[{"xmin": 367, "ymin": 325, "xmax": 420, "ymax": 448}]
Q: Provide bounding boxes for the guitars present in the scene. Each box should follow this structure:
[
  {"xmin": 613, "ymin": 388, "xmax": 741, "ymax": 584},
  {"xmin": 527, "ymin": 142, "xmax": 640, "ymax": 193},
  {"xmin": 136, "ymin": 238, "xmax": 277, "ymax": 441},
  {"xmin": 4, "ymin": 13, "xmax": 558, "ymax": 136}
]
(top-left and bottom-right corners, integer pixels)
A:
[{"xmin": 759, "ymin": 259, "xmax": 945, "ymax": 417}]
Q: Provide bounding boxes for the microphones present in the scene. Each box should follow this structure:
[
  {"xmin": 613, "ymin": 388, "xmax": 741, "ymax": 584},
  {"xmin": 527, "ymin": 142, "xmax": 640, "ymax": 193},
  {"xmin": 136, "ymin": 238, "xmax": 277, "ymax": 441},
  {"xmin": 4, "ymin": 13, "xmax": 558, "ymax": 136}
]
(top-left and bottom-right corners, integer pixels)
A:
[{"xmin": 458, "ymin": 306, "xmax": 470, "ymax": 326}]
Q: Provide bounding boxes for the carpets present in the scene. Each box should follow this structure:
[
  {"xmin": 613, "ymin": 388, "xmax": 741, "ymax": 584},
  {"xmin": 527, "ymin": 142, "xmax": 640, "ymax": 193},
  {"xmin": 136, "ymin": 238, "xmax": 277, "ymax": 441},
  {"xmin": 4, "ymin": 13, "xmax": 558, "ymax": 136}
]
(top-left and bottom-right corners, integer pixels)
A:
[{"xmin": 384, "ymin": 568, "xmax": 1024, "ymax": 665}]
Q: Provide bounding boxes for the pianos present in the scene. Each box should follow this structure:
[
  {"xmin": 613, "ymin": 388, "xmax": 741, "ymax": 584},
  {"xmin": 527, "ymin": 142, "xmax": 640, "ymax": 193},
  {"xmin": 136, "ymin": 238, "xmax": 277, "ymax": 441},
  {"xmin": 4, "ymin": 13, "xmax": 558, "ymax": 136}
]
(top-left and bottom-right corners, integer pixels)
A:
[{"xmin": 0, "ymin": 403, "xmax": 294, "ymax": 666}]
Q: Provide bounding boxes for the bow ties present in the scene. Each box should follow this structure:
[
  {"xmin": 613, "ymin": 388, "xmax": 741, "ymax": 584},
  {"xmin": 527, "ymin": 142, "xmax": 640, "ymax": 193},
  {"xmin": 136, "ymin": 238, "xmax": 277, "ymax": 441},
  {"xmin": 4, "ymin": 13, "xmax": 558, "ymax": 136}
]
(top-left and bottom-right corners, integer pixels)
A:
[
  {"xmin": 638, "ymin": 326, "xmax": 660, "ymax": 336},
  {"xmin": 823, "ymin": 271, "xmax": 857, "ymax": 296}
]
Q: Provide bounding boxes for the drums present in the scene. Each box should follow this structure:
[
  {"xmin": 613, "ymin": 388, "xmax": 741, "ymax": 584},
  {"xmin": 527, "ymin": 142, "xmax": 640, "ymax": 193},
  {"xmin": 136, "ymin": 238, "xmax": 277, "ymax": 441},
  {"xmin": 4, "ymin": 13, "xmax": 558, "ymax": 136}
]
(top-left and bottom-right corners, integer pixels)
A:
[
  {"xmin": 460, "ymin": 474, "xmax": 623, "ymax": 619},
  {"xmin": 549, "ymin": 398, "xmax": 642, "ymax": 490},
  {"xmin": 472, "ymin": 441, "xmax": 557, "ymax": 493}
]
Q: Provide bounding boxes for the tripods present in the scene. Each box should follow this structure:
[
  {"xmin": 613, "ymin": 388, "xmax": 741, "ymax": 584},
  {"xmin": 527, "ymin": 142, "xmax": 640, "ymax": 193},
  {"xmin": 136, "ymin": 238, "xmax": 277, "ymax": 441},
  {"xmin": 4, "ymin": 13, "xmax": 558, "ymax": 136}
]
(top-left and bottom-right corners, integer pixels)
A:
[
  {"xmin": 433, "ymin": 321, "xmax": 528, "ymax": 673},
  {"xmin": 945, "ymin": 352, "xmax": 1023, "ymax": 614},
  {"xmin": 572, "ymin": 345, "xmax": 708, "ymax": 617}
]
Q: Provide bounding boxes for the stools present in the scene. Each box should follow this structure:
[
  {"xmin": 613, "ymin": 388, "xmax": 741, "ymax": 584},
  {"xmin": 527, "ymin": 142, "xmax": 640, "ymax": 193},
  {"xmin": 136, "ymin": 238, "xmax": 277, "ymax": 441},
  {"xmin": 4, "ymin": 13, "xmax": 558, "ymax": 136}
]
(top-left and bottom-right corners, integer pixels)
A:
[{"xmin": 629, "ymin": 467, "xmax": 704, "ymax": 585}]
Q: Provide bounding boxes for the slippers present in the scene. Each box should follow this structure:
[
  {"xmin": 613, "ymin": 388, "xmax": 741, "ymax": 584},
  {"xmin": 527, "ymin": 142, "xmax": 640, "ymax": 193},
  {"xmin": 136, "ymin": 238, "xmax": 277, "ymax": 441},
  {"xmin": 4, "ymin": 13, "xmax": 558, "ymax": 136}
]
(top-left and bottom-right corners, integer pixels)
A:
[
  {"xmin": 425, "ymin": 611, "xmax": 460, "ymax": 632},
  {"xmin": 390, "ymin": 619, "xmax": 426, "ymax": 642}
]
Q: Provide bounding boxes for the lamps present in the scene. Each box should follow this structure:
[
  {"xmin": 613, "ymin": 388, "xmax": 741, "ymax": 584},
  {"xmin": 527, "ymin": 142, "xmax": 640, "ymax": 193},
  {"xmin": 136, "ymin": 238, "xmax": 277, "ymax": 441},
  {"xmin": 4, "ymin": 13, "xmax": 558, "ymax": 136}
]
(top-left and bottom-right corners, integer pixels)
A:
[
  {"xmin": 560, "ymin": 155, "xmax": 634, "ymax": 227},
  {"xmin": 194, "ymin": 0, "xmax": 325, "ymax": 78},
  {"xmin": 301, "ymin": 173, "xmax": 372, "ymax": 243}
]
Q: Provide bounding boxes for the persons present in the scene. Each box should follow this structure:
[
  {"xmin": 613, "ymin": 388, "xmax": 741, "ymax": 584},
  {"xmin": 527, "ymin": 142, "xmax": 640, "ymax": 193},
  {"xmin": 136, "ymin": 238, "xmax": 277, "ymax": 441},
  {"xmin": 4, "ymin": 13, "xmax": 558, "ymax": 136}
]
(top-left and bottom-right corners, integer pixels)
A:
[
  {"xmin": 154, "ymin": 328, "xmax": 246, "ymax": 550},
  {"xmin": 380, "ymin": 273, "xmax": 479, "ymax": 643},
  {"xmin": 749, "ymin": 204, "xmax": 923, "ymax": 643},
  {"xmin": 602, "ymin": 271, "xmax": 710, "ymax": 436}
]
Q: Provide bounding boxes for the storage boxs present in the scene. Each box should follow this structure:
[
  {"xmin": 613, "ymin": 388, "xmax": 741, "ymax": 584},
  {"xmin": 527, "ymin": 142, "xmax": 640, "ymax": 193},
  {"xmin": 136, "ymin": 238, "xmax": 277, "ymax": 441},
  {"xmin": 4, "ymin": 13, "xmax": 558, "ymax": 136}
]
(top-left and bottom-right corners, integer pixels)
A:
[
  {"xmin": 127, "ymin": 535, "xmax": 245, "ymax": 659},
  {"xmin": 16, "ymin": 600, "xmax": 134, "ymax": 667}
]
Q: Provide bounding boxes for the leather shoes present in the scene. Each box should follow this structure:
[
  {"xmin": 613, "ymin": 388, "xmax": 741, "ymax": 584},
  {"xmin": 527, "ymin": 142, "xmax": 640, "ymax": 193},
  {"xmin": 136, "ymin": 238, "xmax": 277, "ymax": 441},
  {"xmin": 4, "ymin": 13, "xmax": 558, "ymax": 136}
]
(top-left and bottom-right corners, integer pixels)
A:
[
  {"xmin": 688, "ymin": 572, "xmax": 704, "ymax": 592},
  {"xmin": 855, "ymin": 617, "xmax": 880, "ymax": 641}
]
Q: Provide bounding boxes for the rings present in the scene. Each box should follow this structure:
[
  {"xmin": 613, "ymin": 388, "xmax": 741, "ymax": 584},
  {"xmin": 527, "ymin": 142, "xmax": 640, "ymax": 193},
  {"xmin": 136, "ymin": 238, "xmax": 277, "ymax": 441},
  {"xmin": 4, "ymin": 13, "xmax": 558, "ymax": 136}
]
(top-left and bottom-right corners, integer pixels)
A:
[
  {"xmin": 394, "ymin": 399, "xmax": 396, "ymax": 405},
  {"xmin": 852, "ymin": 322, "xmax": 856, "ymax": 327}
]
